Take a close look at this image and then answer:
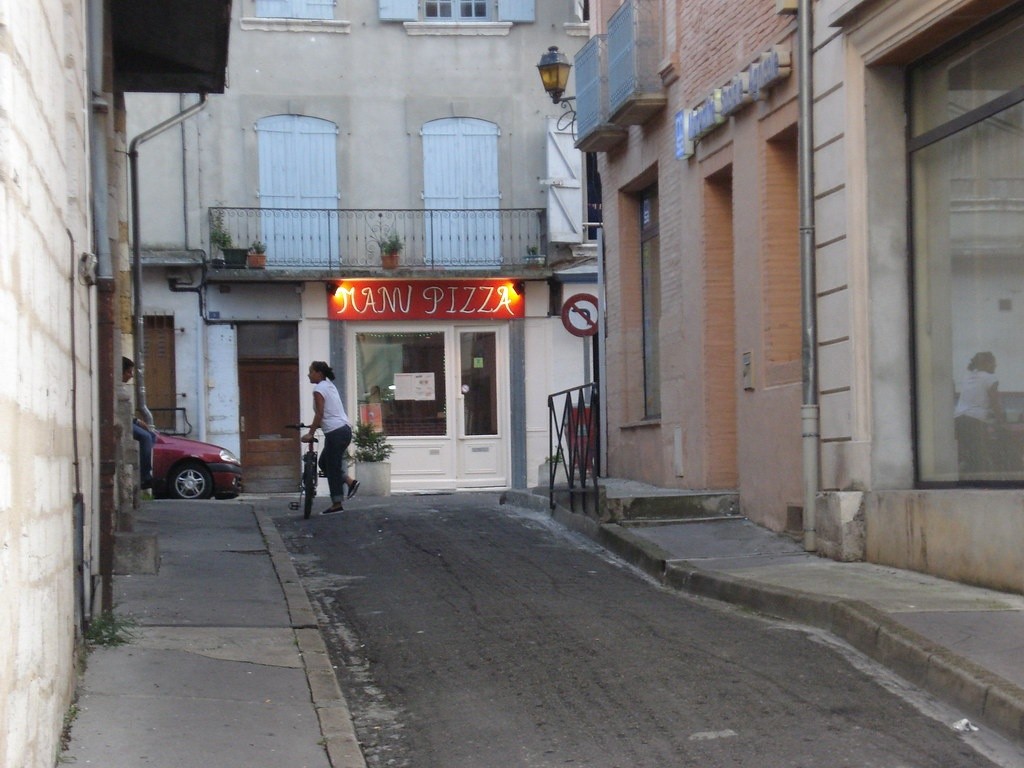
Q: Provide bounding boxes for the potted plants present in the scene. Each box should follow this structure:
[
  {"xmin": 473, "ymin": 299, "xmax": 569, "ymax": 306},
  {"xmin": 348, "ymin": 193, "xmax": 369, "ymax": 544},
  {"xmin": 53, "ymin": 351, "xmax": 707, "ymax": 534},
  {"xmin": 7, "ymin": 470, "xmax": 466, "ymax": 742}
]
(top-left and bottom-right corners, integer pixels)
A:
[
  {"xmin": 246, "ymin": 242, "xmax": 268, "ymax": 269},
  {"xmin": 214, "ymin": 230, "xmax": 250, "ymax": 268},
  {"xmin": 347, "ymin": 425, "xmax": 393, "ymax": 495},
  {"xmin": 379, "ymin": 235, "xmax": 404, "ymax": 267},
  {"xmin": 524, "ymin": 245, "xmax": 546, "ymax": 267}
]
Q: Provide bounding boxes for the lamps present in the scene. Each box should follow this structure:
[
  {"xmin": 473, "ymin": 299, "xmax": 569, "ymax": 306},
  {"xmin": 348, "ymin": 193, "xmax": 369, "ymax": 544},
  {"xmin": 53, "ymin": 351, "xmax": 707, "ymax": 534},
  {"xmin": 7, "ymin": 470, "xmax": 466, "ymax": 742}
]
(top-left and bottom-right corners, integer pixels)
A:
[
  {"xmin": 326, "ymin": 283, "xmax": 340, "ymax": 296},
  {"xmin": 536, "ymin": 45, "xmax": 575, "ymax": 102},
  {"xmin": 513, "ymin": 282, "xmax": 524, "ymax": 295}
]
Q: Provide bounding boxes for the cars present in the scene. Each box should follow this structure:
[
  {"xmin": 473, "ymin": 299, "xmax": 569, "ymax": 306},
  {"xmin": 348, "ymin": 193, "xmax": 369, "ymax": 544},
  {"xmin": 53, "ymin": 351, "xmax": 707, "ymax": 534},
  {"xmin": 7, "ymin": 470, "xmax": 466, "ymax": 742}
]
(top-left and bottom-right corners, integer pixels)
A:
[{"xmin": 133, "ymin": 417, "xmax": 243, "ymax": 500}]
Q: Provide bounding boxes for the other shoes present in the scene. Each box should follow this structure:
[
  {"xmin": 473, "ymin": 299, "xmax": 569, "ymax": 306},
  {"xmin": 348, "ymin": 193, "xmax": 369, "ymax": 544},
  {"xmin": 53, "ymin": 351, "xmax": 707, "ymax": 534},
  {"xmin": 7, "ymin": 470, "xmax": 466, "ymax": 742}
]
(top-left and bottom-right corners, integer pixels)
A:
[
  {"xmin": 140, "ymin": 477, "xmax": 166, "ymax": 490},
  {"xmin": 319, "ymin": 505, "xmax": 345, "ymax": 515},
  {"xmin": 347, "ymin": 480, "xmax": 360, "ymax": 499}
]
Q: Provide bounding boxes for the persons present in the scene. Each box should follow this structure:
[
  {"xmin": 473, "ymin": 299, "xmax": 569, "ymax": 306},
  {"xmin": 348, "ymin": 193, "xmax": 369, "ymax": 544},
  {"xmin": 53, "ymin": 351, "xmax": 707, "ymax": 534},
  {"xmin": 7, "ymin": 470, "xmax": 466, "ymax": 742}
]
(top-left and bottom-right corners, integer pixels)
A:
[
  {"xmin": 302, "ymin": 361, "xmax": 360, "ymax": 516},
  {"xmin": 122, "ymin": 356, "xmax": 161, "ymax": 491}
]
